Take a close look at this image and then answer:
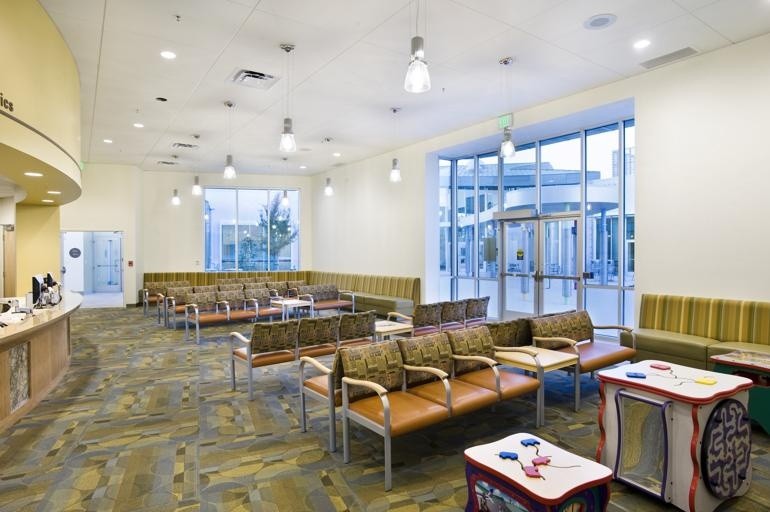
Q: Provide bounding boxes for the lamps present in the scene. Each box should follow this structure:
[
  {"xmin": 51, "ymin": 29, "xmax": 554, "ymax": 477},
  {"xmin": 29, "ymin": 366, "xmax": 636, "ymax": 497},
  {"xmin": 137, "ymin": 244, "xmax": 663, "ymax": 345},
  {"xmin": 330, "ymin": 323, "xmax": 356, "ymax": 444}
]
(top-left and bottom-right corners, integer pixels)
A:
[
  {"xmin": 223, "ymin": 101, "xmax": 237, "ymax": 182},
  {"xmin": 323, "ymin": 138, "xmax": 333, "ymax": 198},
  {"xmin": 279, "ymin": 44, "xmax": 296, "ymax": 155},
  {"xmin": 404, "ymin": 0, "xmax": 432, "ymax": 96},
  {"xmin": 499, "ymin": 56, "xmax": 516, "ymax": 159},
  {"xmin": 171, "ymin": 155, "xmax": 181, "ymax": 207},
  {"xmin": 389, "ymin": 107, "xmax": 401, "ymax": 183},
  {"xmin": 282, "ymin": 158, "xmax": 289, "ymax": 209},
  {"xmin": 191, "ymin": 135, "xmax": 202, "ymax": 197}
]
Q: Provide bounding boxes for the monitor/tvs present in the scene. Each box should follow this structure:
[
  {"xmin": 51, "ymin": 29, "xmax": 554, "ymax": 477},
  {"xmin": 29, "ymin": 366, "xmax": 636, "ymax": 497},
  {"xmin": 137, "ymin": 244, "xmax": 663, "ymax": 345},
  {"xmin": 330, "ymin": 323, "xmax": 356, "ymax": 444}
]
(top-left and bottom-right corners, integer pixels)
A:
[
  {"xmin": 32, "ymin": 273, "xmax": 44, "ymax": 309},
  {"xmin": 47, "ymin": 273, "xmax": 54, "ymax": 287}
]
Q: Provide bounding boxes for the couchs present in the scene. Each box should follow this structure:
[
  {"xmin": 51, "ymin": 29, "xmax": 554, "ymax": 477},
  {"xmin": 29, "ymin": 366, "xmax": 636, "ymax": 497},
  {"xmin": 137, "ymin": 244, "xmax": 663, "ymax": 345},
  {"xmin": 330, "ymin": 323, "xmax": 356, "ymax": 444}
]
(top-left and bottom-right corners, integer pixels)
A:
[
  {"xmin": 143, "ymin": 271, "xmax": 421, "ymax": 321},
  {"xmin": 619, "ymin": 292, "xmax": 770, "ymax": 371}
]
